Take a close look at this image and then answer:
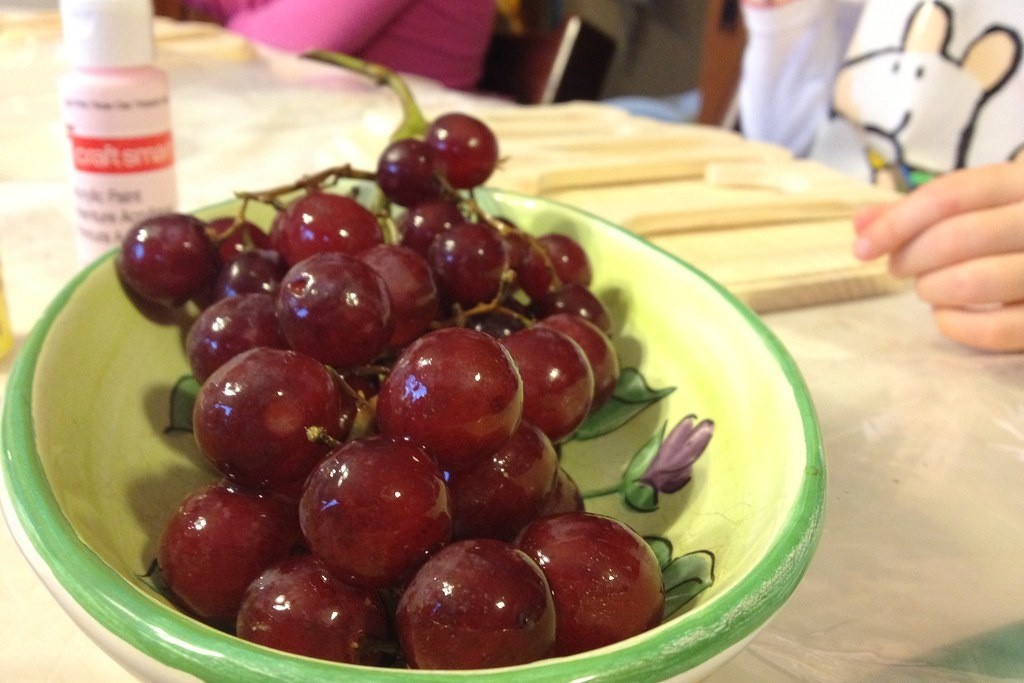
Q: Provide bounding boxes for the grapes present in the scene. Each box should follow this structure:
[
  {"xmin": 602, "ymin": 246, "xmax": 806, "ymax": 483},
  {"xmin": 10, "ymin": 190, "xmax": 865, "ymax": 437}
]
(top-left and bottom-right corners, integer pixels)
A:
[{"xmin": 117, "ymin": 112, "xmax": 666, "ymax": 669}]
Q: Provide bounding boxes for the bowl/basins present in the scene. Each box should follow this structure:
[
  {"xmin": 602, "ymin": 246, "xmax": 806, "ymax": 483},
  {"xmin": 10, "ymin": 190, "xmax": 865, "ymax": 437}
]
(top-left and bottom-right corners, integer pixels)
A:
[{"xmin": 2, "ymin": 183, "xmax": 827, "ymax": 683}]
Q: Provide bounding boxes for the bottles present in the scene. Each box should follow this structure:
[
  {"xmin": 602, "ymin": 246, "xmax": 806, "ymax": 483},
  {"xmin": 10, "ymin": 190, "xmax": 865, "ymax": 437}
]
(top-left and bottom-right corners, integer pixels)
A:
[{"xmin": 59, "ymin": 0, "xmax": 177, "ymax": 256}]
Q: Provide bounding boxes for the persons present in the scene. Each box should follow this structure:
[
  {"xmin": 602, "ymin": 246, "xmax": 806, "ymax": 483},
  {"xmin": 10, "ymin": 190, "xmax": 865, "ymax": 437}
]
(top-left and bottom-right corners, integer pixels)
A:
[
  {"xmin": 190, "ymin": 0, "xmax": 497, "ymax": 90},
  {"xmin": 738, "ymin": 0, "xmax": 1024, "ymax": 353}
]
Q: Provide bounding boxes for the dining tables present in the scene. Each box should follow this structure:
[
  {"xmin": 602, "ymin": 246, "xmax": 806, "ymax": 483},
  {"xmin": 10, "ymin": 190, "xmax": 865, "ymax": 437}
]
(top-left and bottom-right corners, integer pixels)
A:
[{"xmin": 4, "ymin": 1, "xmax": 1024, "ymax": 681}]
